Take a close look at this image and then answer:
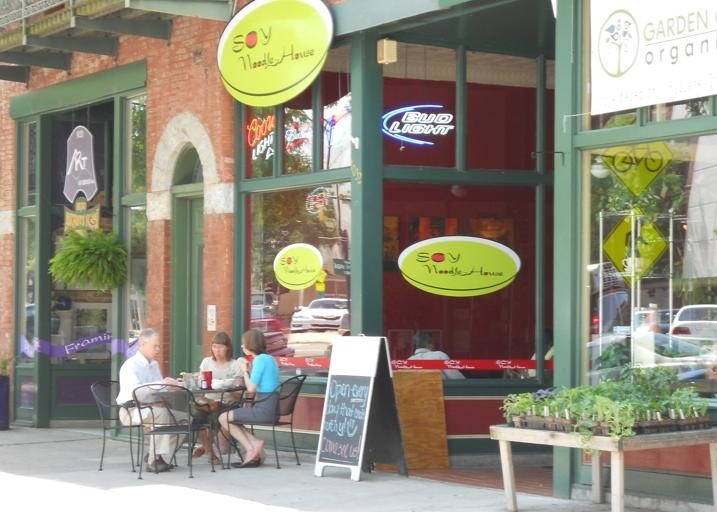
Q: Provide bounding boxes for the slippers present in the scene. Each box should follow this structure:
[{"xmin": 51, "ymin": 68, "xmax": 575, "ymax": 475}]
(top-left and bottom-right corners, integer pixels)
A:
[{"xmin": 192, "ymin": 447, "xmax": 222, "ymax": 465}]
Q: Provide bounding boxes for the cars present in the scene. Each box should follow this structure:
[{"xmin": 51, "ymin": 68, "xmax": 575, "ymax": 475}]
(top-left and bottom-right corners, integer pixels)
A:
[
  {"xmin": 290, "ymin": 298, "xmax": 349, "ymax": 333},
  {"xmin": 586, "ymin": 287, "xmax": 717, "ymax": 398},
  {"xmin": 250, "ymin": 292, "xmax": 295, "ymax": 357}
]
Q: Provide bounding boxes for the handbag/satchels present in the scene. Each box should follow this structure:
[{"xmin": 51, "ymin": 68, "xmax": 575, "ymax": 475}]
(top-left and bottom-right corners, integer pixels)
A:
[{"xmin": 194, "ymin": 396, "xmax": 218, "ymax": 412}]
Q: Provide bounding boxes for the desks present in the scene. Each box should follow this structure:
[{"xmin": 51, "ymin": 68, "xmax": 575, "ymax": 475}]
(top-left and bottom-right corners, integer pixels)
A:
[{"xmin": 491, "ymin": 425, "xmax": 717, "ymax": 512}]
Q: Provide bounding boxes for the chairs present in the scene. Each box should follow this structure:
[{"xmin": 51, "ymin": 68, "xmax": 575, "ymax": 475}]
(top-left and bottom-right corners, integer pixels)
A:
[{"xmin": 88, "ymin": 373, "xmax": 308, "ymax": 481}]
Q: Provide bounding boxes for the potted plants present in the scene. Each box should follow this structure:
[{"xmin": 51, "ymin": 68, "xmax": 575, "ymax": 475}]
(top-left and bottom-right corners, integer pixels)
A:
[{"xmin": 500, "ymin": 364, "xmax": 715, "ymax": 457}]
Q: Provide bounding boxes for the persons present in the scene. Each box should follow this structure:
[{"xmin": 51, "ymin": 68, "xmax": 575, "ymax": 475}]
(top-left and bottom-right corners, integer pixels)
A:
[
  {"xmin": 116, "ymin": 327, "xmax": 193, "ymax": 472},
  {"xmin": 218, "ymin": 329, "xmax": 282, "ymax": 468},
  {"xmin": 192, "ymin": 332, "xmax": 247, "ymax": 465}
]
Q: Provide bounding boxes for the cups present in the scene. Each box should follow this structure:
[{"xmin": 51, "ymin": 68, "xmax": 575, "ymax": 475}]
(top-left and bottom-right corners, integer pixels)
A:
[
  {"xmin": 183, "ymin": 373, "xmax": 192, "ymax": 389},
  {"xmin": 203, "ymin": 371, "xmax": 237, "ymax": 390}
]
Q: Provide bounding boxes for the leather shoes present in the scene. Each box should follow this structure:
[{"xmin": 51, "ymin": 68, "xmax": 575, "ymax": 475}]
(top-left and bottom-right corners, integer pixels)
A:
[{"xmin": 144, "ymin": 453, "xmax": 174, "ymax": 473}]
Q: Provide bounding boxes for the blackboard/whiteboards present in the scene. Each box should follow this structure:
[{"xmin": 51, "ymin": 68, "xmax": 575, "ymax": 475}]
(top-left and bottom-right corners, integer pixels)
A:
[{"xmin": 317, "ymin": 336, "xmax": 382, "ymax": 468}]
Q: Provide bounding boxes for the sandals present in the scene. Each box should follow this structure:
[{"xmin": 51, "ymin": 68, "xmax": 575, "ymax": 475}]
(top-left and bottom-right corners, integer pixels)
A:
[{"xmin": 230, "ymin": 458, "xmax": 261, "ymax": 468}]
